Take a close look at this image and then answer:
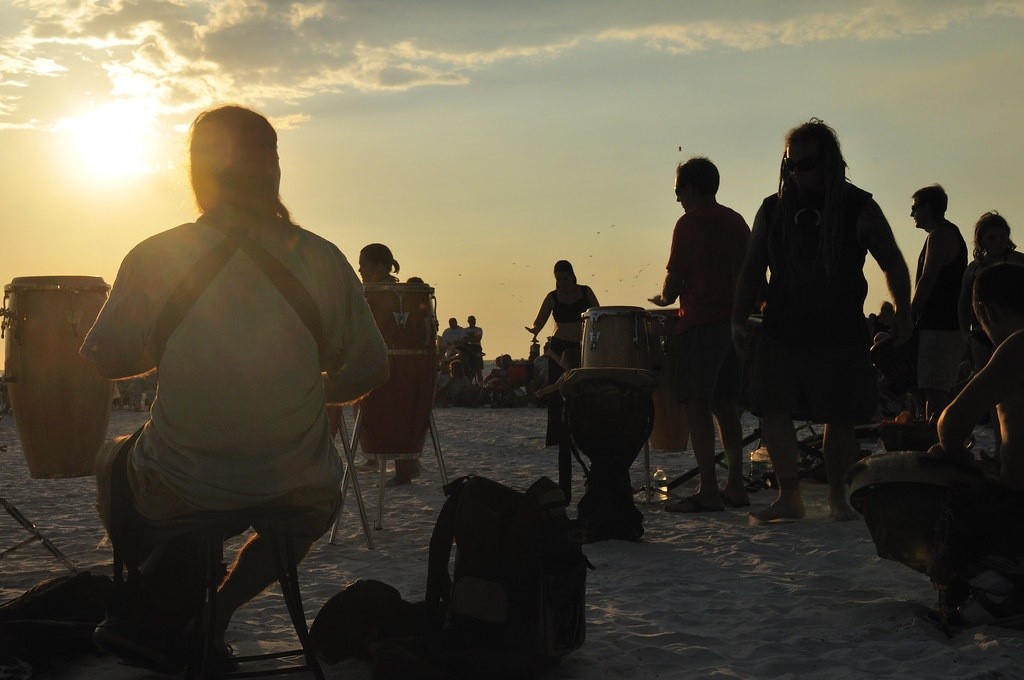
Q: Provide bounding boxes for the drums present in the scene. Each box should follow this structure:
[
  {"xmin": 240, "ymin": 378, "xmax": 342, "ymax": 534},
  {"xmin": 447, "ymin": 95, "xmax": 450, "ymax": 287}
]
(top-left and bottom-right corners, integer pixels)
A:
[
  {"xmin": 841, "ymin": 449, "xmax": 993, "ymax": 575},
  {"xmin": 746, "ymin": 313, "xmax": 764, "ymax": 331},
  {"xmin": 354, "ymin": 282, "xmax": 439, "ymax": 461},
  {"xmin": 323, "ymin": 400, "xmax": 343, "ymax": 439},
  {"xmin": 646, "ymin": 309, "xmax": 692, "ymax": 454},
  {"xmin": 535, "ymin": 366, "xmax": 655, "ymax": 544},
  {"xmin": 869, "ymin": 320, "xmax": 920, "ymax": 397},
  {"xmin": 580, "ymin": 306, "xmax": 656, "ymax": 370},
  {"xmin": 0, "ymin": 275, "xmax": 110, "ymax": 481},
  {"xmin": 507, "ymin": 362, "xmax": 531, "ymax": 386}
]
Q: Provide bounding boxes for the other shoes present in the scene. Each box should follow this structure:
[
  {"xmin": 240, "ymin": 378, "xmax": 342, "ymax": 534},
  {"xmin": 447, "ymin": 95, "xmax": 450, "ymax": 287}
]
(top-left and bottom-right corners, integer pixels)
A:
[{"xmin": 177, "ymin": 626, "xmax": 239, "ymax": 673}]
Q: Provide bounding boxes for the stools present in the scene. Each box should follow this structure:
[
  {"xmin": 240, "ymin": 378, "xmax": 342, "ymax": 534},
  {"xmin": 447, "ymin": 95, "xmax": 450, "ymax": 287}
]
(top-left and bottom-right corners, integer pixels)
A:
[{"xmin": 172, "ymin": 508, "xmax": 323, "ymax": 680}]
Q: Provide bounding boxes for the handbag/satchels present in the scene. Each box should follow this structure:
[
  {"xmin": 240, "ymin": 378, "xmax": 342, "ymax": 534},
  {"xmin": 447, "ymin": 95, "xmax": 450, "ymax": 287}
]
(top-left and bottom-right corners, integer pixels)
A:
[{"xmin": 306, "ymin": 579, "xmax": 401, "ymax": 665}]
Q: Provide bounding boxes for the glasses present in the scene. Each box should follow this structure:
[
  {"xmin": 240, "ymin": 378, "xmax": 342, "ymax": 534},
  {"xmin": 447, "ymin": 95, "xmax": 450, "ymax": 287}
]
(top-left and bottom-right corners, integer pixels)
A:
[{"xmin": 784, "ymin": 156, "xmax": 824, "ymax": 173}]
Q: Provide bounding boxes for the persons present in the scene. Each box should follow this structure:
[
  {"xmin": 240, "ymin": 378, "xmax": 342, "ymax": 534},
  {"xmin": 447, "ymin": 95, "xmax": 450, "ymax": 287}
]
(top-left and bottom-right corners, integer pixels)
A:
[{"xmin": 81, "ymin": 106, "xmax": 1024, "ymax": 650}]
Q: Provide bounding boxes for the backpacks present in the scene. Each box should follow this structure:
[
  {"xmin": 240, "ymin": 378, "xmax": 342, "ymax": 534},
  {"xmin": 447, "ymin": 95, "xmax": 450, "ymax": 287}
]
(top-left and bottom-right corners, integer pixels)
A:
[{"xmin": 425, "ymin": 472, "xmax": 596, "ymax": 680}]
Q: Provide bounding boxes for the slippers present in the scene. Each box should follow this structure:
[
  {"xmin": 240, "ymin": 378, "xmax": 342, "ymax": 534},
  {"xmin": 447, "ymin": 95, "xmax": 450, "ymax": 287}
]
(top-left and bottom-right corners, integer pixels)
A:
[
  {"xmin": 665, "ymin": 496, "xmax": 725, "ymax": 513},
  {"xmin": 722, "ymin": 493, "xmax": 751, "ymax": 507},
  {"xmin": 947, "ymin": 594, "xmax": 1024, "ymax": 631}
]
